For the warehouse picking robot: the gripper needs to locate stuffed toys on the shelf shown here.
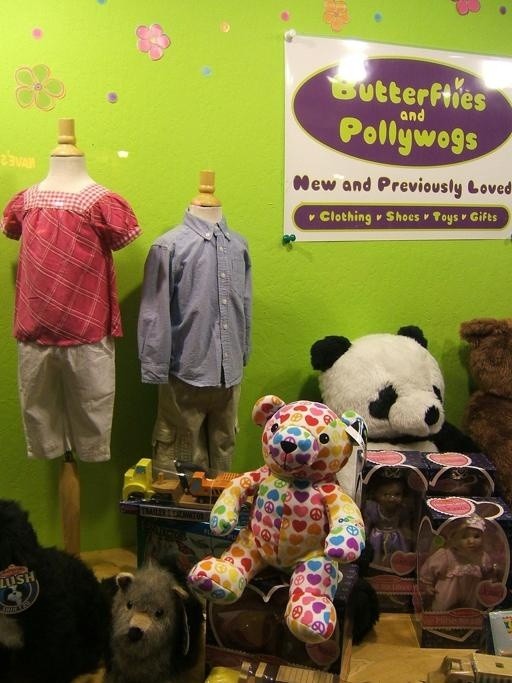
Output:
[97,560,206,682]
[308,324,479,458]
[458,319,510,522]
[0,500,110,683]
[186,395,367,646]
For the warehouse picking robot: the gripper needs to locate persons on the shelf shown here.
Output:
[356,448,512,643]
[2,120,141,557]
[139,170,253,483]
